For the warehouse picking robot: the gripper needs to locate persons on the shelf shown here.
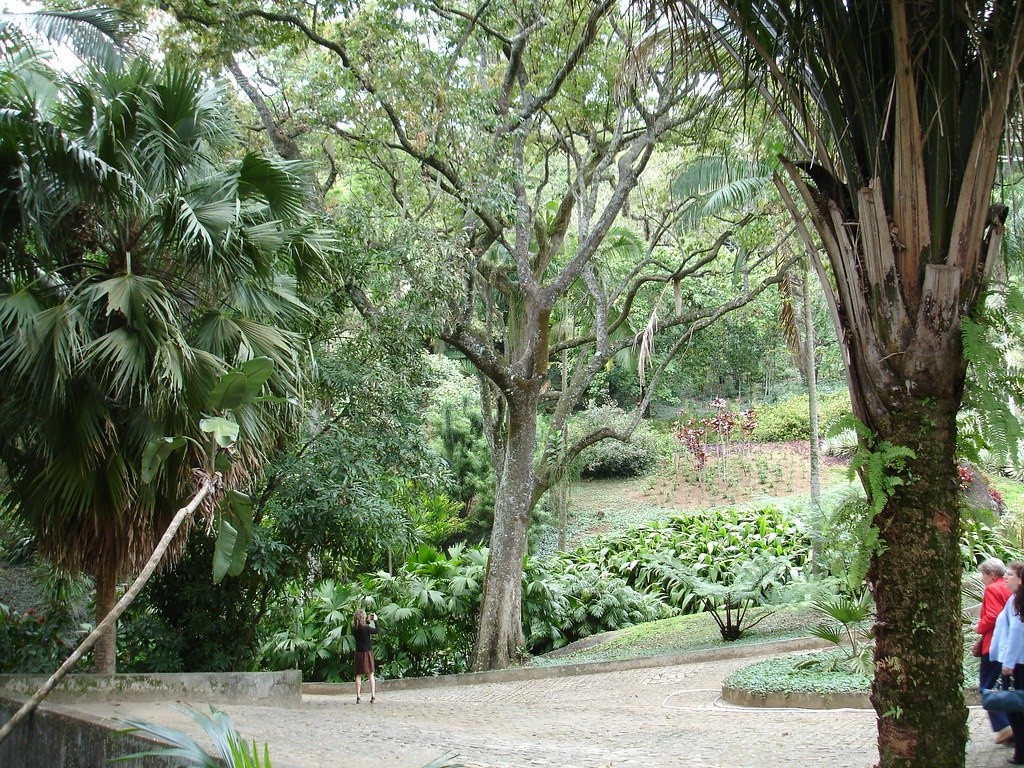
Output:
[351,609,380,705]
[982,690,1024,764]
[988,561,1024,747]
[972,558,1014,712]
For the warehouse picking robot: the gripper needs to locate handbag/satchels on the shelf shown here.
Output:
[973,636,982,657]
[979,674,1024,712]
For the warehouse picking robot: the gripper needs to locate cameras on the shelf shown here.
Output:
[371,615,374,620]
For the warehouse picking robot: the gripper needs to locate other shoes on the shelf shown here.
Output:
[357,697,362,704]
[1007,756,1024,764]
[370,697,375,703]
[995,726,1013,744]
